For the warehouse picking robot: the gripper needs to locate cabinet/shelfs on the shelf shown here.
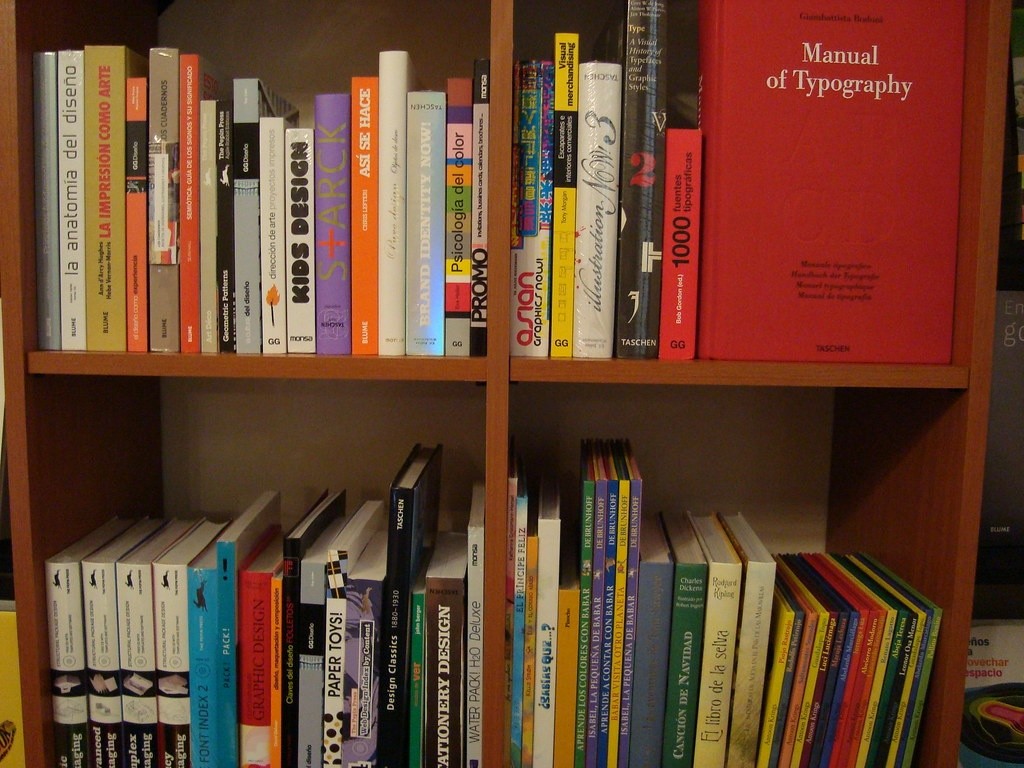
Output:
[0,0,1016,768]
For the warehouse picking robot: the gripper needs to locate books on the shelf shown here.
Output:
[628,509,778,768]
[44,487,388,768]
[576,440,642,768]
[697,1,967,363]
[31,45,314,354]
[507,434,580,768]
[514,33,621,360]
[956,620,1024,768]
[381,442,484,768]
[615,0,702,359]
[313,50,491,356]
[756,549,943,768]
[0,600,27,767]
